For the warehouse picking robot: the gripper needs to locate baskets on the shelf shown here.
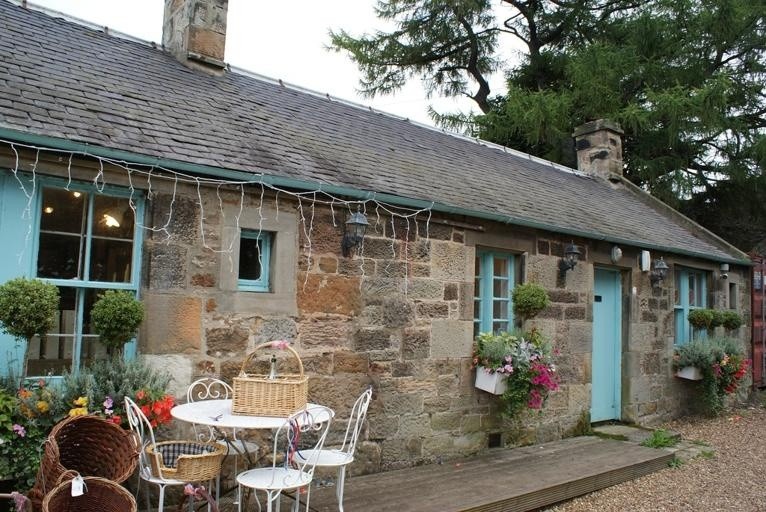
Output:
[232,342,308,417]
[26,414,141,512]
[146,440,226,481]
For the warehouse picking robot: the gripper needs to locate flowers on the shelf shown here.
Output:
[471,333,562,419]
[674,337,752,415]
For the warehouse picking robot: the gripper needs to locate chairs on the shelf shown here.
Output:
[282,388,374,512]
[125,395,221,511]
[235,405,333,512]
[187,378,259,504]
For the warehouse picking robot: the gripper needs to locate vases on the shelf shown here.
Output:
[475,364,509,395]
[675,366,705,381]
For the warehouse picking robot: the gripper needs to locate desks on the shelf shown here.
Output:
[170,398,335,512]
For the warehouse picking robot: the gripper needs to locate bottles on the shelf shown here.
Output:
[267,354,279,379]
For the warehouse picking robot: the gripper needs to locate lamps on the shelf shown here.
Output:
[640,249,650,273]
[103,200,132,229]
[612,245,623,264]
[651,257,669,286]
[341,211,369,258]
[719,264,730,280]
[561,240,581,276]
